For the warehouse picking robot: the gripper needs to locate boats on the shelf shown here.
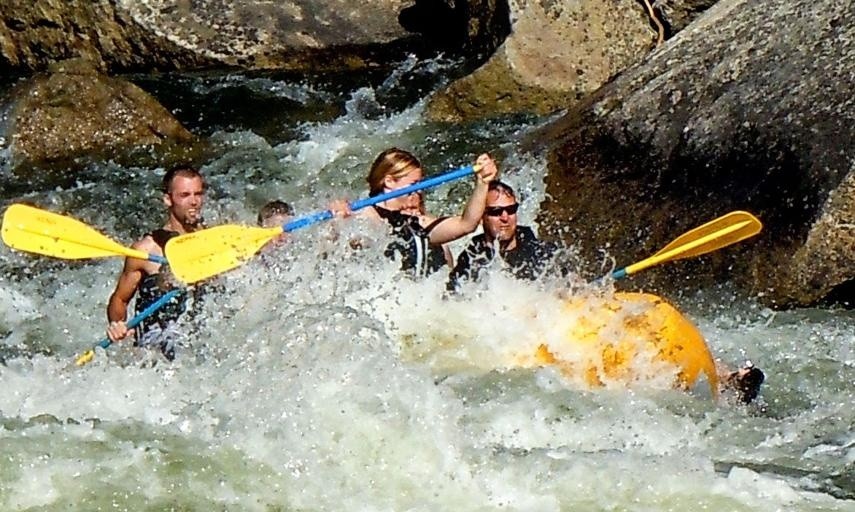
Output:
[417,292,717,405]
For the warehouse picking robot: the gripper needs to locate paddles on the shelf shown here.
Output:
[1,204,168,265]
[587,212,762,289]
[165,162,485,282]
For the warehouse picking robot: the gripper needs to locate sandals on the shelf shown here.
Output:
[724,364,764,403]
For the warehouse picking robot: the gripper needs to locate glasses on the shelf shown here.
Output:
[485,201,519,216]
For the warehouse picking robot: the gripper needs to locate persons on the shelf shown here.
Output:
[325,148,492,283]
[106,167,208,345]
[719,364,764,410]
[256,199,294,255]
[447,179,572,298]
